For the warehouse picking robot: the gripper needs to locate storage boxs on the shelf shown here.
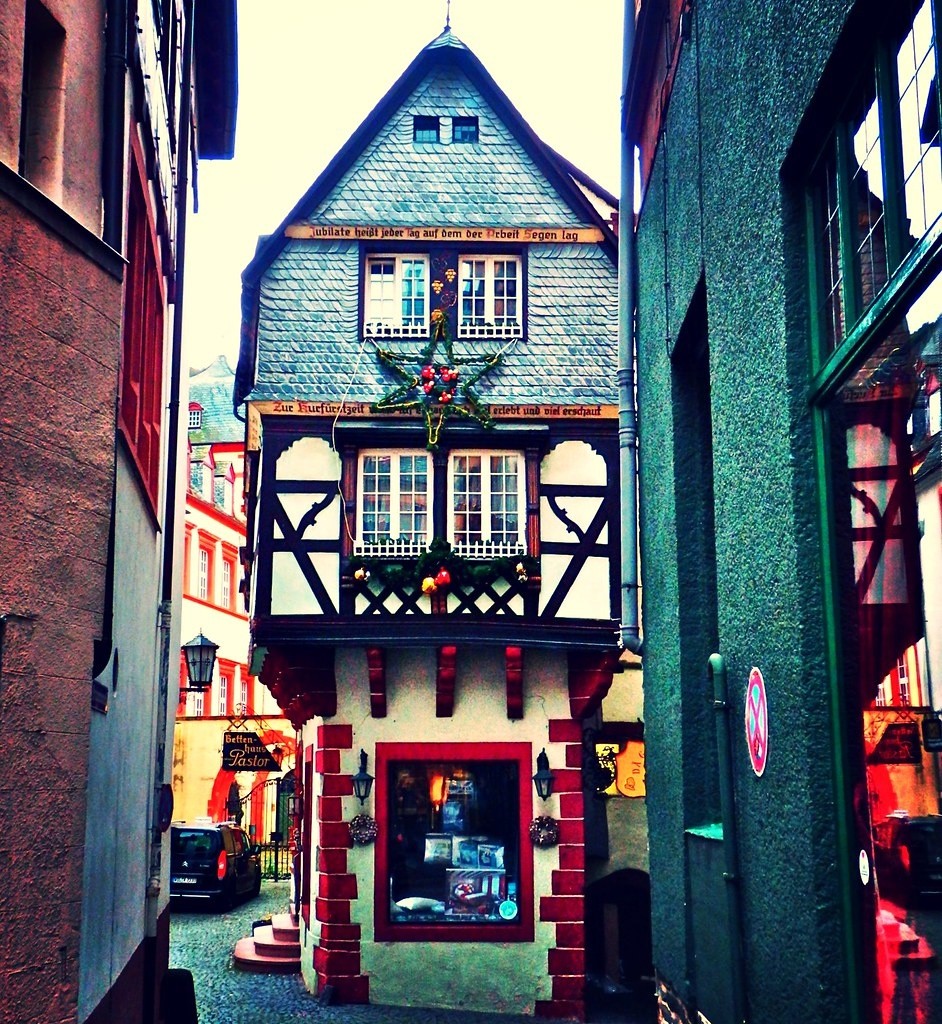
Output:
[424,833,509,920]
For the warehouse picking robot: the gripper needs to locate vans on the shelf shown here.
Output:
[171,822,261,913]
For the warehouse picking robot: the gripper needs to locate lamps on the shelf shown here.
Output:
[350,750,376,806]
[180,628,221,694]
[287,792,304,816]
[533,747,556,800]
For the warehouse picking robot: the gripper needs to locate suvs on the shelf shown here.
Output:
[872,812,942,909]
